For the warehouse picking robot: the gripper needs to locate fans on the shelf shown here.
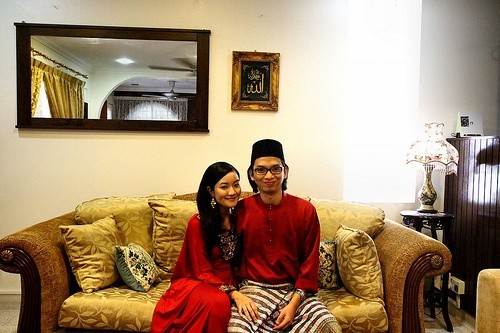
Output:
[149,66,197,76]
[142,81,178,100]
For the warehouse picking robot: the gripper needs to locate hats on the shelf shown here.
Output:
[251,139,284,162]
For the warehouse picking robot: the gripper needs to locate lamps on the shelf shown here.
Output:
[406,122,459,213]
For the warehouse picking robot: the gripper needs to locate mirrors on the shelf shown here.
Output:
[14,23,210,133]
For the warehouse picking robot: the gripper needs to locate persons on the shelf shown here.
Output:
[228,139,342,333]
[151,162,260,333]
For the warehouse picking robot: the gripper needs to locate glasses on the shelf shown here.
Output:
[252,166,285,175]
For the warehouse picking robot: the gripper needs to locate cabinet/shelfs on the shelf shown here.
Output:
[442,136,500,328]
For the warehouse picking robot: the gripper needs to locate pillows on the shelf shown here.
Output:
[148,199,199,278]
[114,242,161,293]
[318,242,339,290]
[75,192,176,256]
[334,224,385,303]
[59,214,123,293]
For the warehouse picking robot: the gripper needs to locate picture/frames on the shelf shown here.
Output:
[231,50,280,111]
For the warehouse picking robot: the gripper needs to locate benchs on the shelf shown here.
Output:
[0,191,452,333]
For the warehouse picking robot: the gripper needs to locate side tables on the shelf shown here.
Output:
[401,210,455,333]
